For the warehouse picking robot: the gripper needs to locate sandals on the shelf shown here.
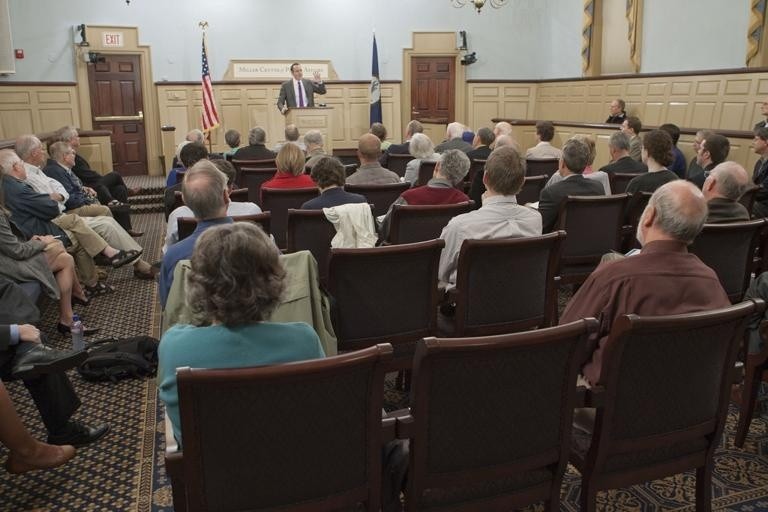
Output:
[107,201,130,213]
[108,249,143,268]
[85,281,116,297]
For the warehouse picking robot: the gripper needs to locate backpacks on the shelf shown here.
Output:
[77,337,160,381]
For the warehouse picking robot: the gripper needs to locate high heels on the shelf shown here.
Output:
[58,320,101,337]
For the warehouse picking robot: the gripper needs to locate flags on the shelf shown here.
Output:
[370,35,382,125]
[200,34,221,141]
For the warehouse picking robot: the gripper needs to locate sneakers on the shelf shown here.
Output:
[134,264,160,280]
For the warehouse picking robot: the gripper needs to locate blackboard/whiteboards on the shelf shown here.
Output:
[0,0,16,74]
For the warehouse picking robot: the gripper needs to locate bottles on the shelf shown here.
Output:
[70,315,83,354]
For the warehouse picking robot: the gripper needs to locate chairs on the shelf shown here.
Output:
[18,282,42,303]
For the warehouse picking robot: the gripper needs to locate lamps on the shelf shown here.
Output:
[451,0,509,13]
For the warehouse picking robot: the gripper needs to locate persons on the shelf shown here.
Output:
[157,123,324,447]
[540,116,767,434]
[0,126,160,475]
[605,99,626,124]
[277,62,327,115]
[301,134,399,211]
[373,120,563,315]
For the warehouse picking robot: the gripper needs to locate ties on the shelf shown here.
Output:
[298,82,306,108]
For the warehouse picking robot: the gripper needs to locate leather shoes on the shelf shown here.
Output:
[12,343,88,378]
[72,295,91,307]
[6,443,75,474]
[128,229,143,237]
[48,421,110,447]
